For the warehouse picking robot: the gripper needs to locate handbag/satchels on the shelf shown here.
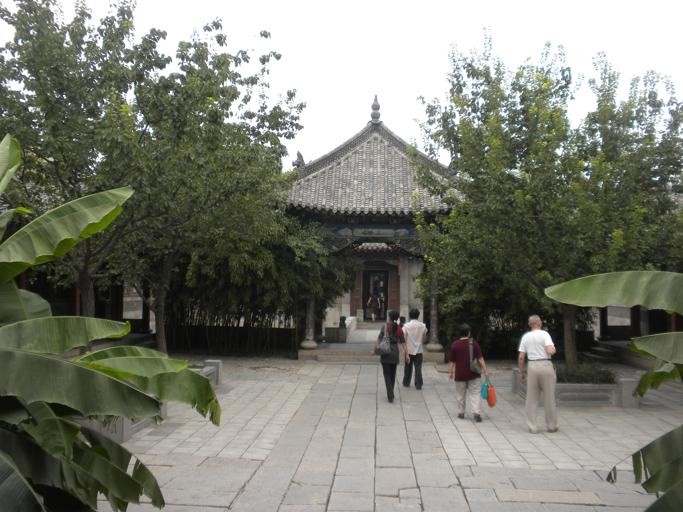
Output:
[374,323,390,355]
[470,361,496,406]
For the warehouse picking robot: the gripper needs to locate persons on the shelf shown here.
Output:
[367,290,381,322]
[446,324,489,422]
[517,314,559,434]
[376,308,428,404]
